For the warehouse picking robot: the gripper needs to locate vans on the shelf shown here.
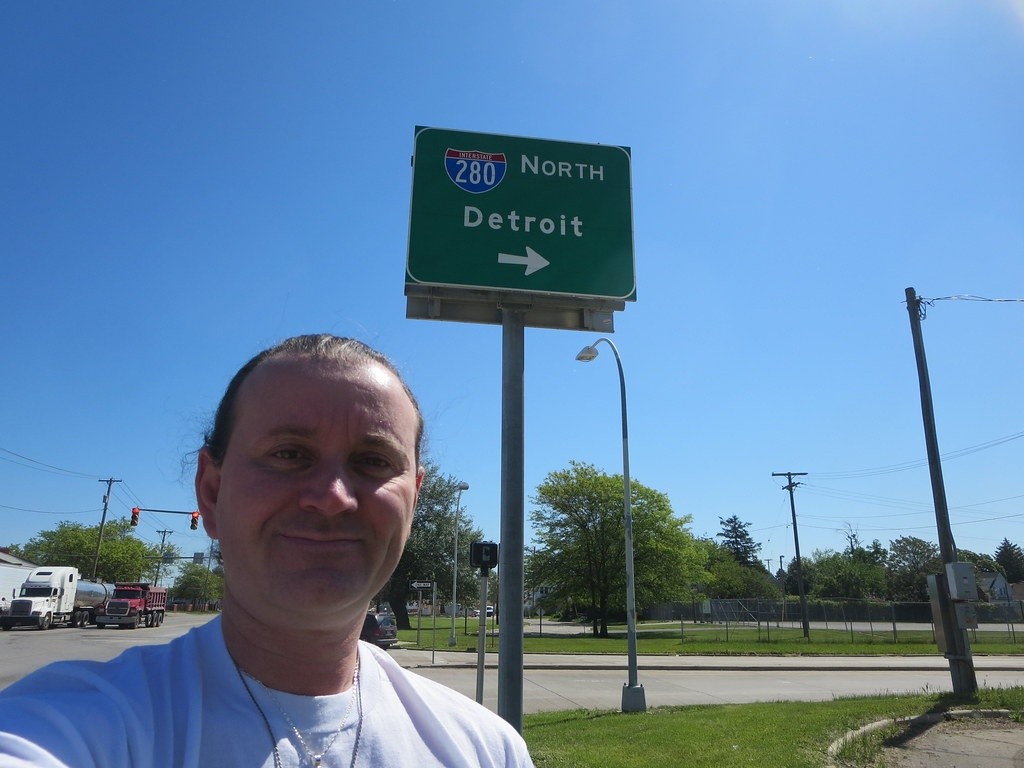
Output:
[487,606,494,616]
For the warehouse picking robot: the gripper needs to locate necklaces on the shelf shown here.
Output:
[227,646,364,768]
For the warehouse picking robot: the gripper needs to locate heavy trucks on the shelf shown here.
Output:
[95,582,166,629]
[0,566,117,631]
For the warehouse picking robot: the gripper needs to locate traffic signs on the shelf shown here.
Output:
[409,579,435,591]
[405,123,637,303]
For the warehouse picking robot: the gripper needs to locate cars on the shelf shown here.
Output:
[471,610,480,617]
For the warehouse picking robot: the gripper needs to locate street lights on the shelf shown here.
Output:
[574,337,649,715]
[449,481,470,646]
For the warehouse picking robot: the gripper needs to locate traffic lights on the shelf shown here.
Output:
[190,511,200,530]
[130,507,140,526]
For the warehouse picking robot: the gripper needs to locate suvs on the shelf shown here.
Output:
[360,612,399,650]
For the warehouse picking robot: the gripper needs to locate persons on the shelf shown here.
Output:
[0,333,535,768]
